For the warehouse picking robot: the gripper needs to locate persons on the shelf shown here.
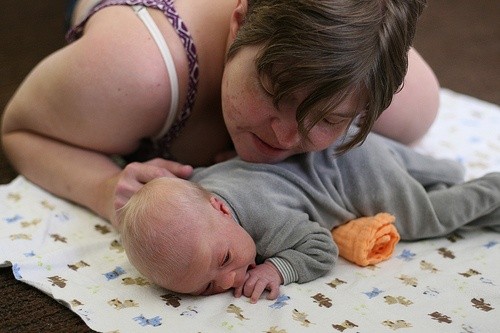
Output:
[0,0,440,227]
[115,126,500,304]
